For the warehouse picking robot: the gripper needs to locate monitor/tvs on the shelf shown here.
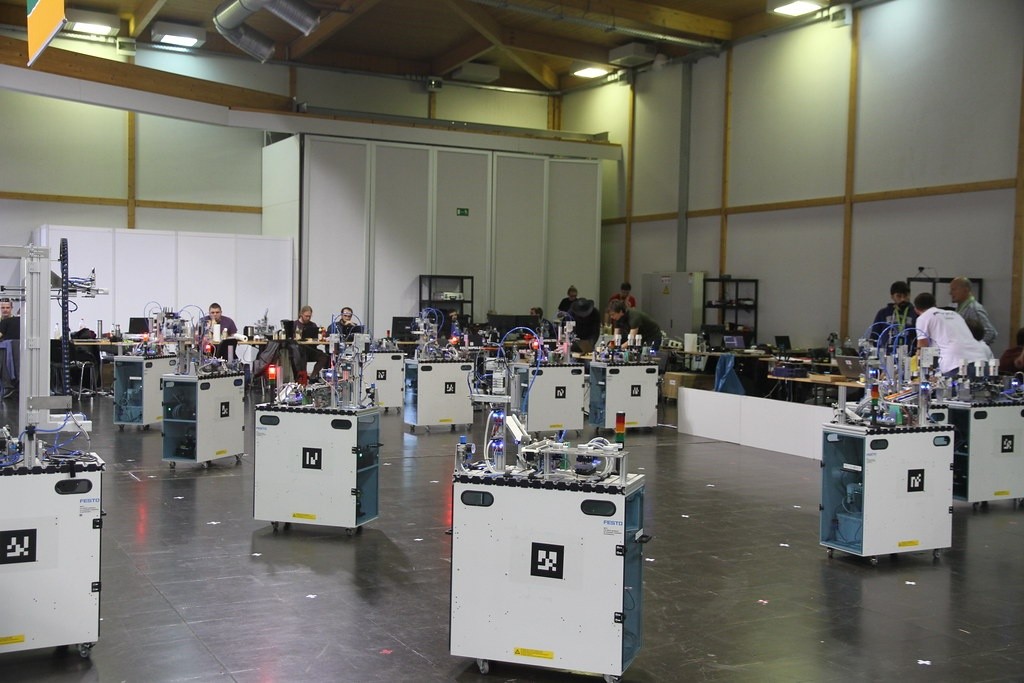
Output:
[342,326,364,341]
[515,315,540,336]
[392,317,415,341]
[775,336,791,350]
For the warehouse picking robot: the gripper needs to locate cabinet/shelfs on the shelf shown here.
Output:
[932,398,1024,509]
[0,348,663,683]
[819,420,955,569]
[418,275,474,333]
[701,279,760,350]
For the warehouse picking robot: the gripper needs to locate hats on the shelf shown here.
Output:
[570,297,594,317]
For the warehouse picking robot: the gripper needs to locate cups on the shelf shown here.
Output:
[244,325,254,340]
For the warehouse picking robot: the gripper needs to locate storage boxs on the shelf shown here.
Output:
[664,371,714,399]
[836,513,862,545]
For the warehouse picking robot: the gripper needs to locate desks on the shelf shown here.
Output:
[75,342,137,356]
[299,341,352,345]
[574,353,594,360]
[237,341,267,347]
[394,340,418,345]
[676,351,771,372]
[505,339,528,347]
[767,375,866,405]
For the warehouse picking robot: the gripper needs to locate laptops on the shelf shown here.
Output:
[298,327,319,341]
[835,355,865,380]
[125,318,152,334]
[724,336,746,350]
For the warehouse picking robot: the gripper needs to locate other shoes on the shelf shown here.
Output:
[310,373,319,379]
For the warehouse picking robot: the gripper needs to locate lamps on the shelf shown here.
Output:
[63,8,120,37]
[150,21,208,49]
[764,0,830,19]
[557,61,613,81]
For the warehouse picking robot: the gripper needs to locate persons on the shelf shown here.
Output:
[0,297,20,399]
[326,307,357,367]
[559,285,600,338]
[196,303,237,357]
[870,276,1024,387]
[604,282,663,351]
[294,306,329,384]
[530,307,557,351]
[449,310,468,337]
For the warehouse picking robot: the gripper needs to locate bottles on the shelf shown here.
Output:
[295,325,301,339]
[96,319,102,338]
[386,330,391,341]
[53,323,60,339]
[110,324,121,339]
[273,328,286,340]
[79,318,85,330]
[318,325,326,341]
[221,327,228,339]
